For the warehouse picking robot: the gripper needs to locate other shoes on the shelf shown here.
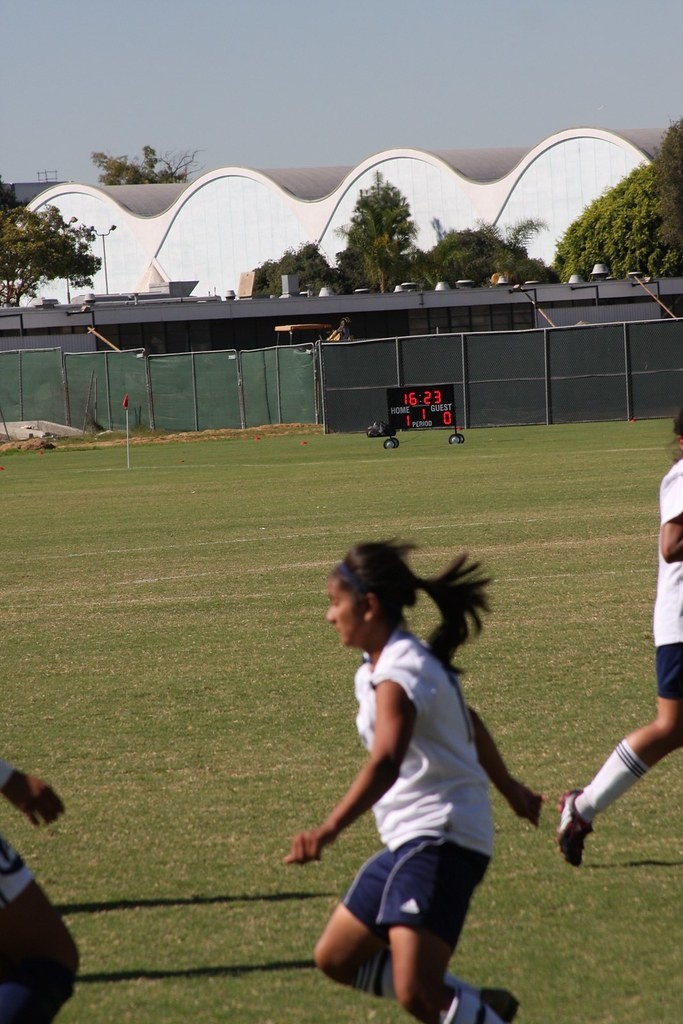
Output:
[480,984,519,1024]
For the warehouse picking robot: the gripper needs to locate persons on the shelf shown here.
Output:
[555,406,683,866]
[283,535,542,1024]
[0,751,81,1024]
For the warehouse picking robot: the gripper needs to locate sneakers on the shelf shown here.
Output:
[556,789,594,867]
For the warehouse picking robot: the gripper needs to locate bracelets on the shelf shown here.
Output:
[492,761,512,792]
